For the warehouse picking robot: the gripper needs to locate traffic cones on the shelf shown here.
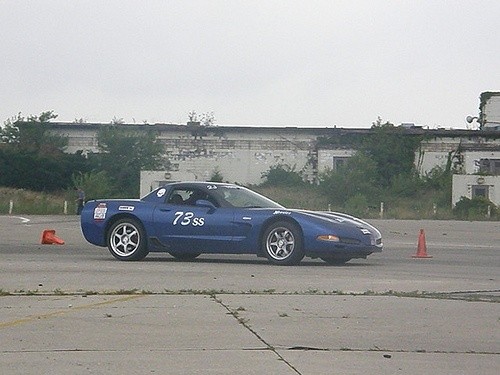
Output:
[40,229,65,246]
[410,228,433,259]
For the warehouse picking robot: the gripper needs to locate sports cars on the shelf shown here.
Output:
[81,181,385,266]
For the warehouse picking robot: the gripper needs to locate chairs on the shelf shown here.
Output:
[169,189,226,207]
